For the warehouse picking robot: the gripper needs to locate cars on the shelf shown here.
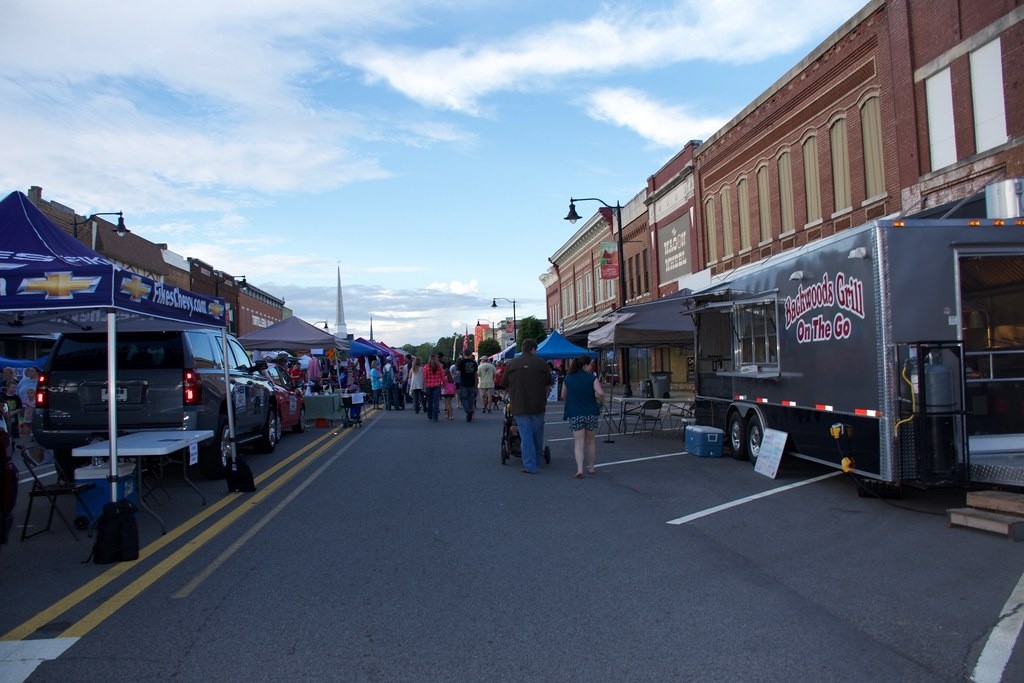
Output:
[261,363,306,443]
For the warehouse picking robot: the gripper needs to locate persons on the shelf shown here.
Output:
[547,362,565,400]
[498,338,554,474]
[319,348,509,423]
[560,355,605,478]
[0,367,39,457]
[286,362,305,389]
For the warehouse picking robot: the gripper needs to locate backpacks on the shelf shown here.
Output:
[382,365,395,388]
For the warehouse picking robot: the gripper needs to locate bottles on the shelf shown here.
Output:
[91,436,101,466]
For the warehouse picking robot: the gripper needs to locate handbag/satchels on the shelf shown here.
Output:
[409,387,415,400]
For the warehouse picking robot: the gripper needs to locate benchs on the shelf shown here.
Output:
[66,347,129,369]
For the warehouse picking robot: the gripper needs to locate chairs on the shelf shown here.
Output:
[16,444,101,542]
[599,400,695,438]
[163,346,184,366]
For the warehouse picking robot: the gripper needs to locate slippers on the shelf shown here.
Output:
[575,473,584,478]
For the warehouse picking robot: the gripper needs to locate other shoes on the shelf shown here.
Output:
[372,405,383,410]
[427,414,439,421]
[482,408,492,414]
[415,407,428,414]
[467,411,474,421]
[388,405,402,412]
[447,415,454,419]
[521,467,526,472]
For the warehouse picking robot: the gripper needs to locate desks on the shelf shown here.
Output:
[72,430,215,536]
[605,397,695,434]
[339,404,364,429]
[302,392,367,426]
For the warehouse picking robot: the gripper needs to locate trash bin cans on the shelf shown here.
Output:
[651,371,672,398]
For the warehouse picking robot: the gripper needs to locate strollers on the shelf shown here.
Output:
[501,402,551,465]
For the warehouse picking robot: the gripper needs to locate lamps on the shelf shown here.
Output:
[969,308,985,329]
[847,246,866,259]
[789,271,804,281]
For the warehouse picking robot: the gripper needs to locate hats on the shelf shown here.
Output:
[437,352,446,357]
[480,355,489,360]
[387,356,393,360]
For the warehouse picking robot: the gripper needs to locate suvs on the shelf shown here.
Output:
[31,323,280,480]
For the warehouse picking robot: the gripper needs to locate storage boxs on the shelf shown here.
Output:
[74,462,141,529]
[684,425,724,458]
[682,421,695,443]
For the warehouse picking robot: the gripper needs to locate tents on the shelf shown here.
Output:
[514,330,599,379]
[311,337,405,404]
[1,189,238,502]
[587,305,695,443]
[235,316,366,421]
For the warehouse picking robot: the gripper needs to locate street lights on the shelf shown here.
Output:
[72,211,132,242]
[564,198,633,399]
[476,319,494,339]
[490,297,516,342]
[215,275,249,298]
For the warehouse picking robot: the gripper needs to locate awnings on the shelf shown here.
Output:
[547,307,613,338]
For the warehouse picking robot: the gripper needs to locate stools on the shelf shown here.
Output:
[365,393,374,405]
